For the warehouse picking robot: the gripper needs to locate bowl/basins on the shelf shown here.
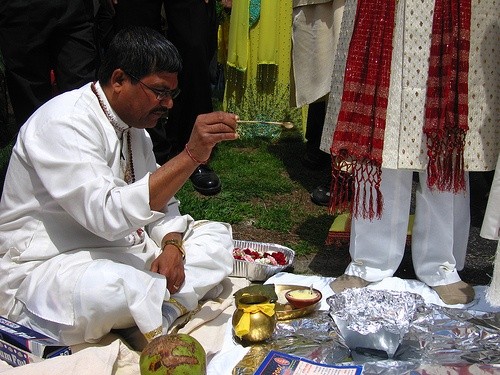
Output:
[285,288,321,308]
[233,284,322,320]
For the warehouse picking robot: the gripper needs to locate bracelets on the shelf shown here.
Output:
[162,240,186,261]
[185,143,208,166]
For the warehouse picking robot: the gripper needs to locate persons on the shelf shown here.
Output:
[1,25,239,351]
[319,1,500,305]
[0,1,500,215]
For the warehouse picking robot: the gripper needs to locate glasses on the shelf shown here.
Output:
[124,70,181,101]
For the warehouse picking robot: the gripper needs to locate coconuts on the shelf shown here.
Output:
[139,309,208,375]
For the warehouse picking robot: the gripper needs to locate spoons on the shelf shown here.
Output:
[236,121,293,128]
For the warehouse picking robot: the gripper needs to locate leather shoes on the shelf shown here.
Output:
[189,162,222,194]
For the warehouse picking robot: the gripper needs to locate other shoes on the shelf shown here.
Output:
[313,176,350,206]
